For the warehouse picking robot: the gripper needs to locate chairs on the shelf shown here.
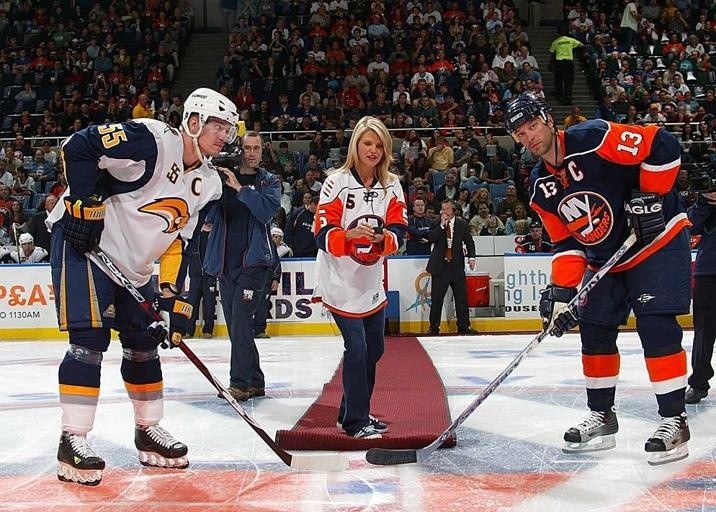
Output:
[1,38,58,232]
[291,140,515,201]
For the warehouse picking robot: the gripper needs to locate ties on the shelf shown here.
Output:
[446,221,452,260]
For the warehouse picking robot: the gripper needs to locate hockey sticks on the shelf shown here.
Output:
[366,232,637,466]
[92,243,350,471]
[13,223,21,264]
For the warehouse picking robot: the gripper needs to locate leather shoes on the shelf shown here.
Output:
[458,328,476,333]
[428,329,438,334]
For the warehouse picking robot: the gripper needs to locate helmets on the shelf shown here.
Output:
[182,87,239,127]
[19,233,33,247]
[504,94,551,135]
[270,227,284,240]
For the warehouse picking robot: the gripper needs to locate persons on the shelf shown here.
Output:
[504,94,692,452]
[201,130,283,402]
[312,115,409,440]
[252,242,281,339]
[44,88,239,470]
[183,210,215,339]
[685,192,716,404]
[425,198,479,336]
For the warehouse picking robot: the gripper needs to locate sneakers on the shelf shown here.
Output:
[337,414,388,439]
[564,407,618,442]
[57,430,105,469]
[135,424,188,458]
[685,387,708,403]
[645,412,690,451]
[218,387,265,400]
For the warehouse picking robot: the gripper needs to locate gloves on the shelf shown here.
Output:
[631,187,665,242]
[63,196,105,254]
[144,292,194,347]
[540,283,578,338]
